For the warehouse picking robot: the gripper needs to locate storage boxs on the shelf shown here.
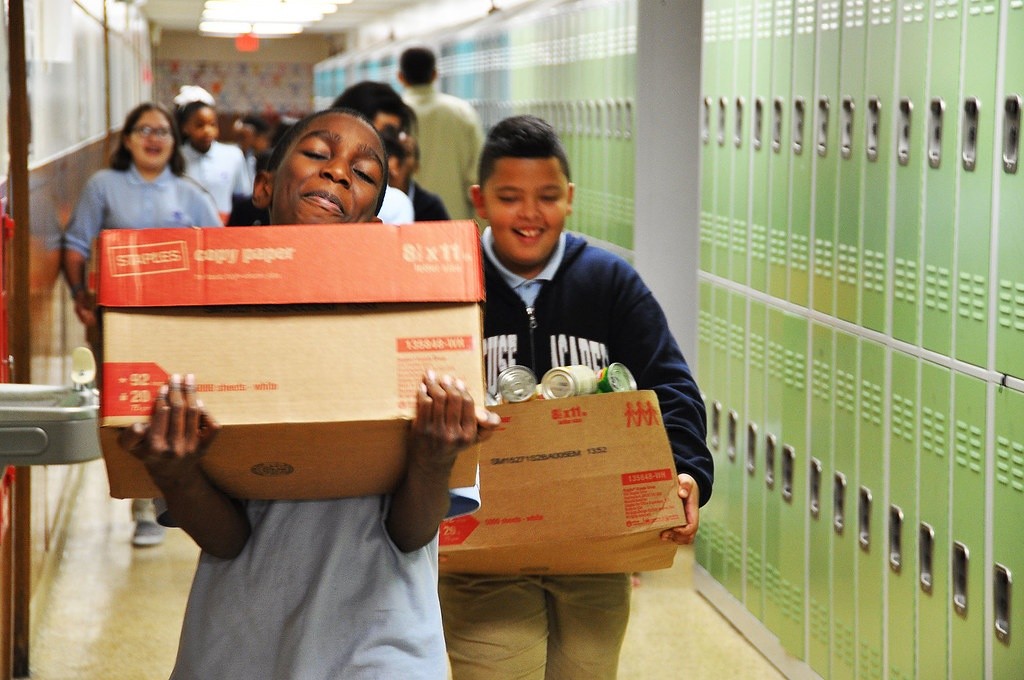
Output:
[85,219,483,500]
[437,389,686,577]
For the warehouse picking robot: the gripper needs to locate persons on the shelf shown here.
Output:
[61,102,228,546]
[230,81,453,225]
[116,106,502,680]
[392,47,485,224]
[170,80,251,227]
[437,115,717,680]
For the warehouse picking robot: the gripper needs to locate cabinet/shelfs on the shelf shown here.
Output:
[690,0,1024,680]
[502,1,637,267]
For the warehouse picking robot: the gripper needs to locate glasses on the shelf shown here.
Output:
[132,125,171,140]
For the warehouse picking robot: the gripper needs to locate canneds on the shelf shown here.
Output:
[496,361,637,404]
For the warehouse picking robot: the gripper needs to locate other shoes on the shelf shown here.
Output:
[132,521,165,546]
